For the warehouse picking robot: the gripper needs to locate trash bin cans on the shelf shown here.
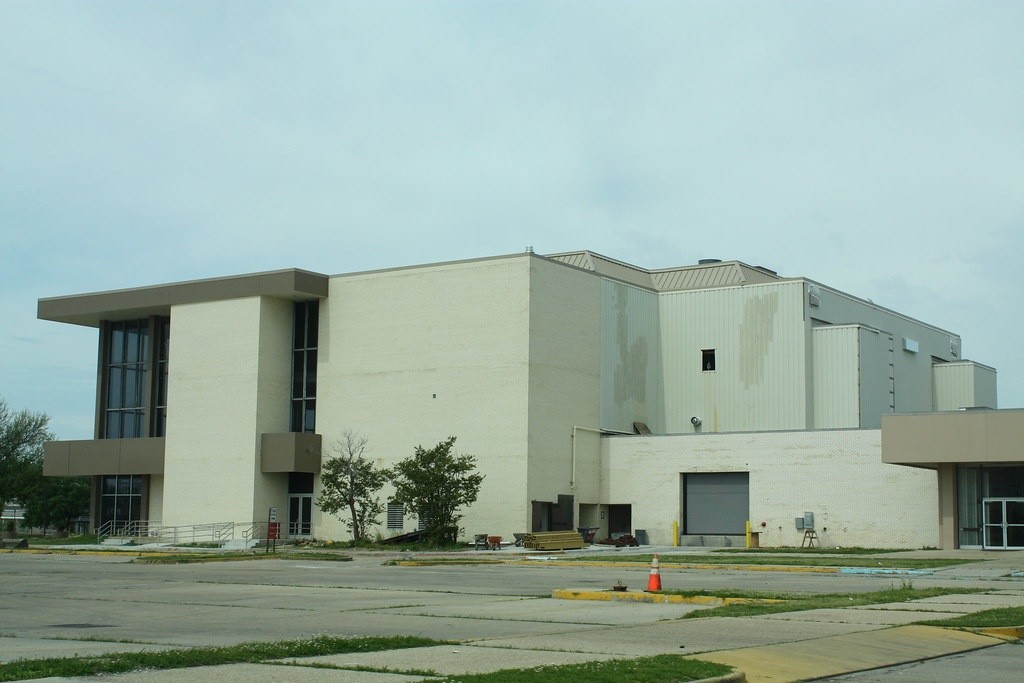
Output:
[269,522,281,539]
[635,529,645,544]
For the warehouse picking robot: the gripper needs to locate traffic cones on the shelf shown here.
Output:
[644,554,662,592]
